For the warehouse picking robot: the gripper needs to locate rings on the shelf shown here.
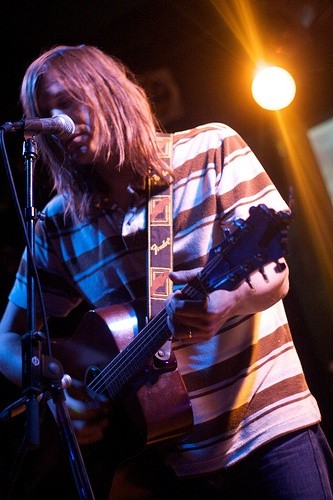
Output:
[189,329,192,339]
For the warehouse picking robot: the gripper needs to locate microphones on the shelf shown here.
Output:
[1,114,75,140]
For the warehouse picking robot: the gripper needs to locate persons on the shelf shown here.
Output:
[0,45,333,500]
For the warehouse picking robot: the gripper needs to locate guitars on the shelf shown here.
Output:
[0,204,294,500]
